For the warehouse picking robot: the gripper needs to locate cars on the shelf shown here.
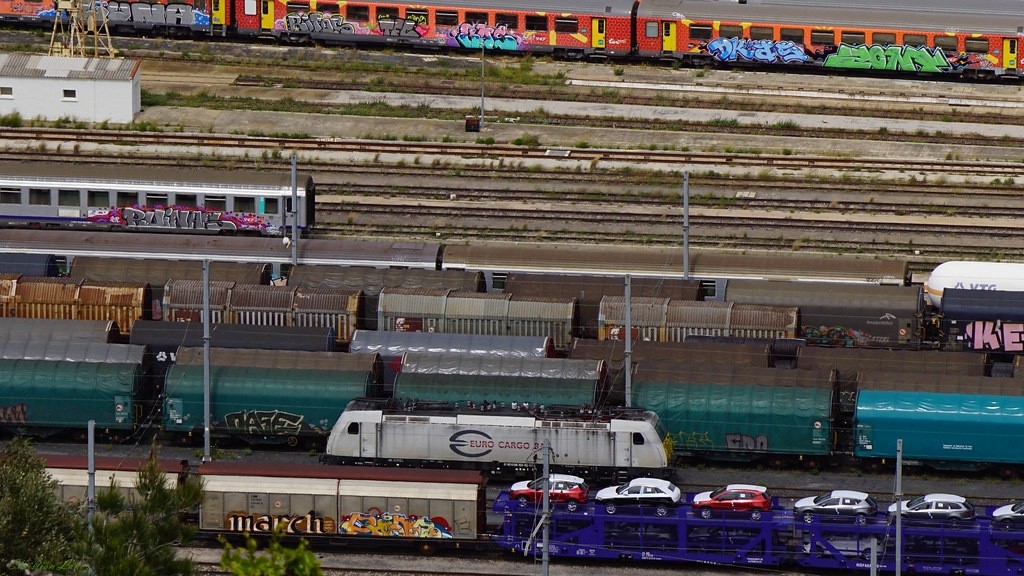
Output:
[803,533,885,562]
[605,522,673,547]
[594,477,681,517]
[992,500,1024,533]
[793,490,878,525]
[688,526,762,555]
[692,484,773,521]
[518,516,579,543]
[905,535,978,566]
[510,474,589,512]
[886,494,976,530]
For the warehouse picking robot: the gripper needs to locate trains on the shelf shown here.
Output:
[0,159,1024,576]
[0,0,1024,85]
